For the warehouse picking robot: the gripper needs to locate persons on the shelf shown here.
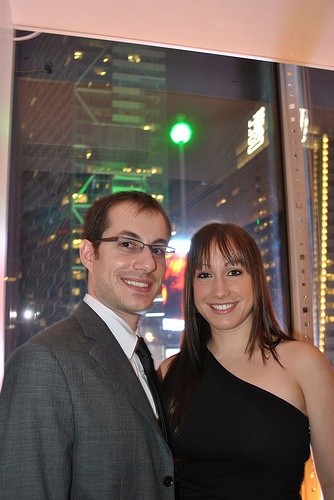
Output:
[0,190,173,499]
[157,223,334,500]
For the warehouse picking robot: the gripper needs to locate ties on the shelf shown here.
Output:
[135,337,168,444]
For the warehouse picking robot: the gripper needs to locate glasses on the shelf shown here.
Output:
[90,236,176,260]
